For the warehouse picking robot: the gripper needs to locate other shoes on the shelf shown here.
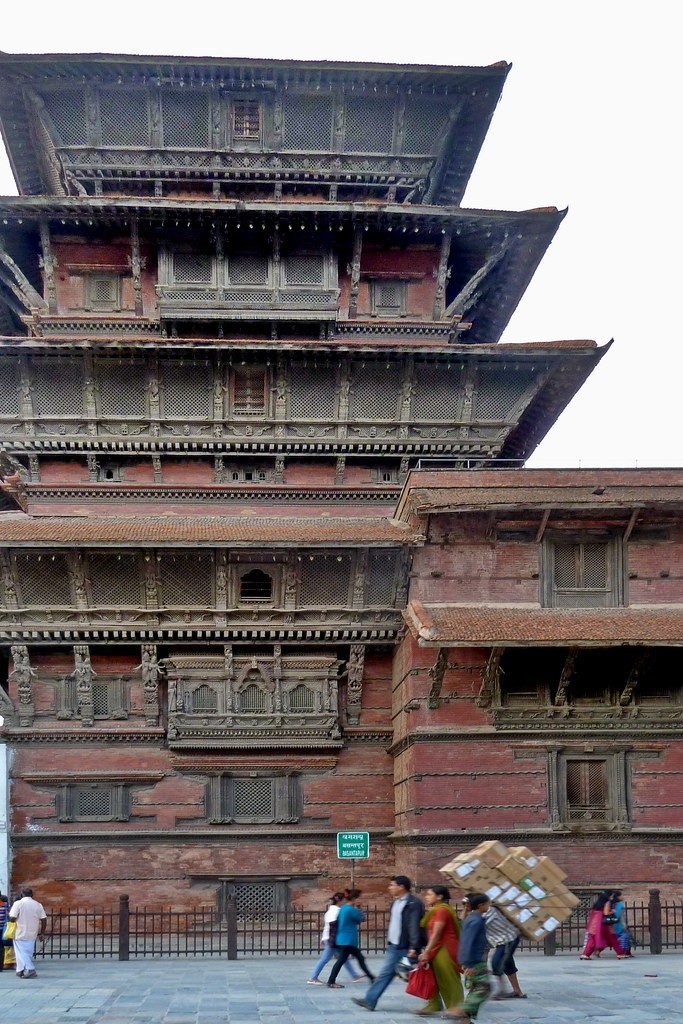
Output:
[499,993,528,1000]
[306,979,326,986]
[442,1008,475,1024]
[15,971,23,977]
[353,975,367,982]
[327,983,345,988]
[580,955,590,960]
[617,954,631,959]
[413,1006,439,1015]
[349,998,375,1012]
[21,969,36,978]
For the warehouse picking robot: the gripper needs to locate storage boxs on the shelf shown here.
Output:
[439,840,580,943]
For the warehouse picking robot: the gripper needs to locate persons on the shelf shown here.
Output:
[135,653,165,686]
[459,895,527,1000]
[446,893,492,1024]
[397,568,408,595]
[426,651,448,686]
[356,562,366,591]
[66,556,91,590]
[560,655,580,681]
[580,890,632,960]
[17,373,478,401]
[348,655,364,688]
[216,569,228,590]
[307,888,378,988]
[9,653,39,686]
[34,228,452,285]
[0,558,15,593]
[9,888,49,979]
[480,656,501,695]
[139,564,162,588]
[417,885,465,1019]
[73,655,97,686]
[0,891,15,972]
[287,565,298,589]
[351,876,425,1011]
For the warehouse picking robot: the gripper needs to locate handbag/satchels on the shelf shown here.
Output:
[3,921,16,938]
[406,961,437,1000]
[328,921,339,949]
[602,913,619,924]
